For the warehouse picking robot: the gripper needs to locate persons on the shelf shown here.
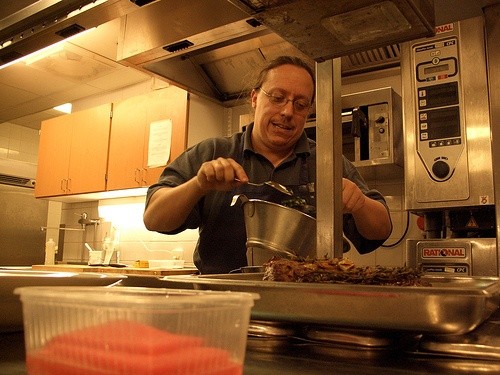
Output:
[143,55,393,275]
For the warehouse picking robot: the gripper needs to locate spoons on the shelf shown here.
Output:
[233,177,295,196]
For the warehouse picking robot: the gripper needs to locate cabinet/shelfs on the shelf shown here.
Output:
[36,85,231,204]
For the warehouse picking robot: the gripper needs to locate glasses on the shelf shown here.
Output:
[259,86,311,111]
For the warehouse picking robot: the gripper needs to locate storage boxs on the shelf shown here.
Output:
[15,285,260,375]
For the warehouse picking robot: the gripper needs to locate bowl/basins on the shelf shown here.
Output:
[230,192,351,266]
